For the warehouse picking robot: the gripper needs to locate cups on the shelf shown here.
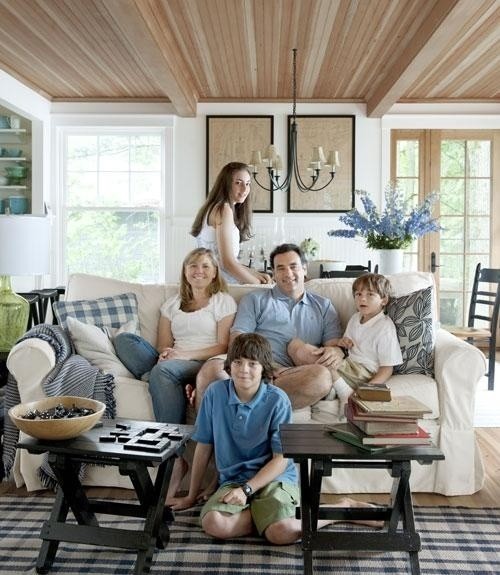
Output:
[7,114,21,130]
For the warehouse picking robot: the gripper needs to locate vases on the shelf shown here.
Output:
[0,274,30,353]
[377,249,404,273]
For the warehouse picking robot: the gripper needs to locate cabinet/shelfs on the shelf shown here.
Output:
[1,106,45,219]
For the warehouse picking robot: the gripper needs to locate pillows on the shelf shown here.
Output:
[382,286,438,375]
[48,293,140,346]
[66,316,135,382]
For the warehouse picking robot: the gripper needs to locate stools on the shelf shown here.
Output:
[33,289,58,326]
[16,293,40,331]
[57,286,65,294]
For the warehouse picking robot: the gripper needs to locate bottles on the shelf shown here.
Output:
[274,218,286,245]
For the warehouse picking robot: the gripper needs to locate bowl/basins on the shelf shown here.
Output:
[6,393,108,441]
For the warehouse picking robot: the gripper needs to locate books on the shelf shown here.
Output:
[325,381,433,455]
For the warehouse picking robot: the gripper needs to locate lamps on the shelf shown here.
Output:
[250,49,339,193]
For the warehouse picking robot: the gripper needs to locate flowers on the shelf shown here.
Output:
[327,180,445,249]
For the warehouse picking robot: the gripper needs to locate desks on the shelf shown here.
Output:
[278,423,445,575]
[14,416,196,575]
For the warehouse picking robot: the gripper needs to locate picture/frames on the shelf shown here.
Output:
[207,114,274,212]
[288,115,355,213]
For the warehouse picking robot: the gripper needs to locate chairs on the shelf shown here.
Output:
[318,264,378,278]
[440,263,500,392]
[346,260,372,271]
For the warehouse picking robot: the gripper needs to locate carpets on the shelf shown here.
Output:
[0,492,500,575]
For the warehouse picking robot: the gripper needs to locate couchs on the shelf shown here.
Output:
[7,271,487,496]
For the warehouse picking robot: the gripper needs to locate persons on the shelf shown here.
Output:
[286,272,403,423]
[191,162,272,285]
[165,333,385,545]
[195,244,342,506]
[113,247,237,502]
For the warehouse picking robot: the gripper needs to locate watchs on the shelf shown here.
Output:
[243,483,253,496]
[340,346,349,360]
